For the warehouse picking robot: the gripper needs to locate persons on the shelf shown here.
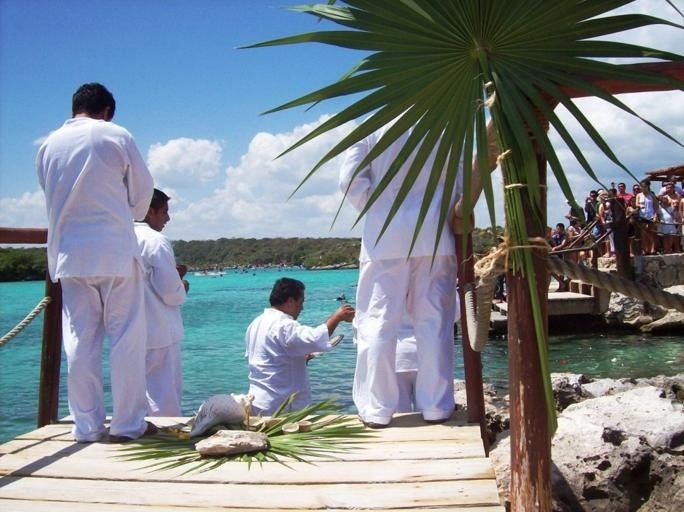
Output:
[129,186,190,417]
[32,81,157,444]
[338,105,465,430]
[494,180,684,302]
[244,275,356,418]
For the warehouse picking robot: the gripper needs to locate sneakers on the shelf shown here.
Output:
[107,421,157,442]
